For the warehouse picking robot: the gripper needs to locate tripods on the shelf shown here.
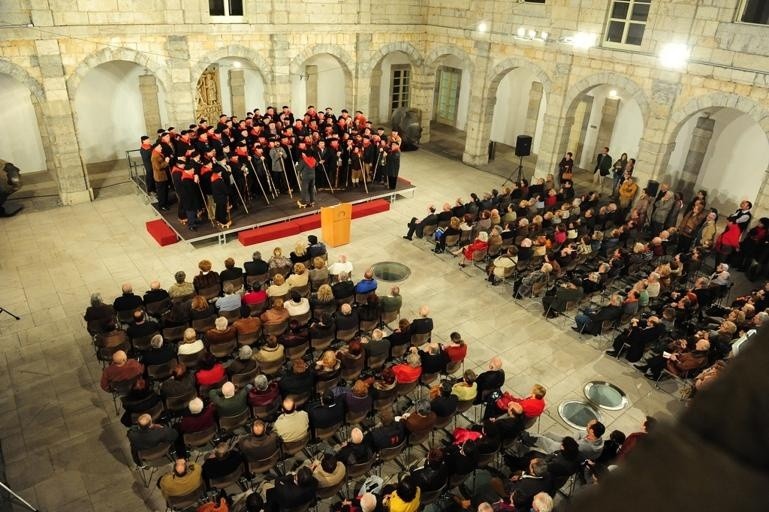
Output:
[502,157,525,187]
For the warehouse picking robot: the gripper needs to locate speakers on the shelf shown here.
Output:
[515,135,532,156]
[647,180,659,197]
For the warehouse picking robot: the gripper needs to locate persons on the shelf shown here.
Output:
[609,153,627,197]
[589,147,612,198]
[570,245,769,405]
[85,234,433,457]
[619,159,635,189]
[406,174,768,319]
[558,152,573,189]
[157,330,656,511]
[141,106,402,230]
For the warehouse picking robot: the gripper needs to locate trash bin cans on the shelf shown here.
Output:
[488,141,497,161]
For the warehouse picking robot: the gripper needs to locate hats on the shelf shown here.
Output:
[140,106,399,173]
